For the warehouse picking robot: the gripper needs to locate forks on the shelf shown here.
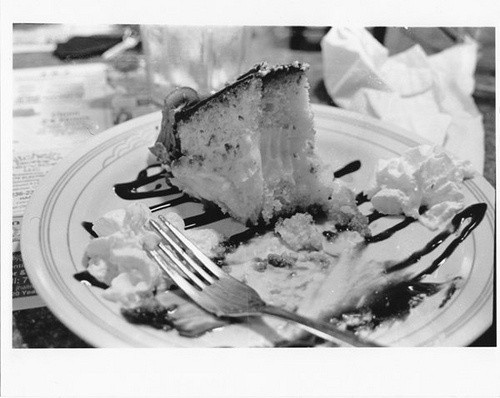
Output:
[142,217,389,349]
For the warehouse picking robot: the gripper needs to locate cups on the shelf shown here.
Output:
[140,26,292,101]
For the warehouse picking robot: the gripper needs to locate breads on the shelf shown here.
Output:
[168,60,328,227]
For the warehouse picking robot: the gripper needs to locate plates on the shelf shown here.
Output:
[19,103,497,347]
